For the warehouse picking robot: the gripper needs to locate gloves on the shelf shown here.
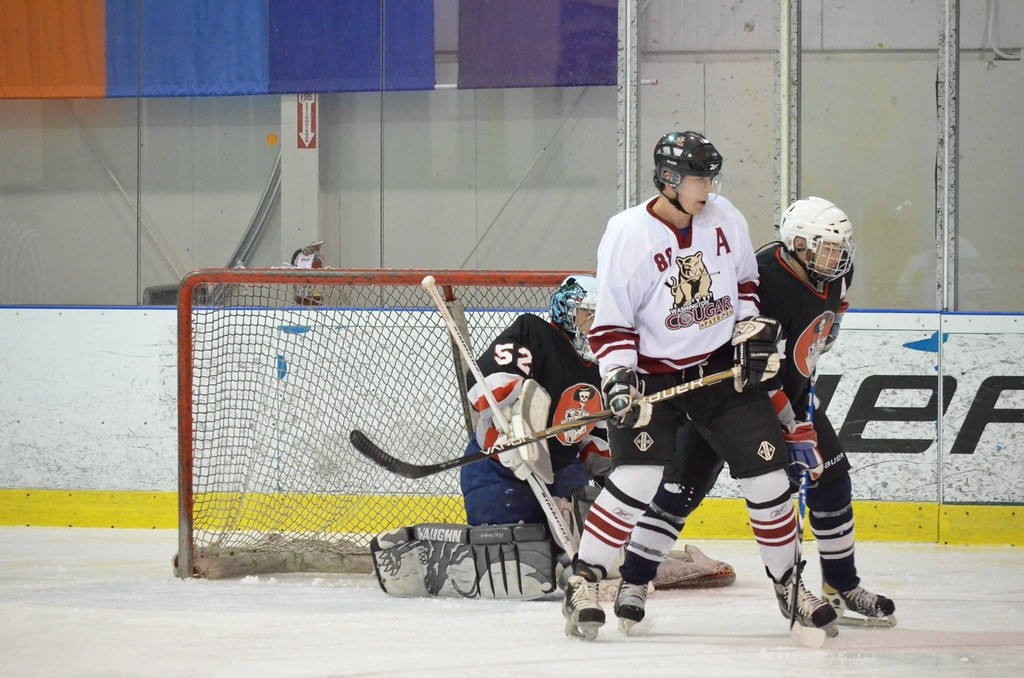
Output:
[732,315,787,393]
[781,420,824,489]
[603,369,654,430]
[820,301,848,354]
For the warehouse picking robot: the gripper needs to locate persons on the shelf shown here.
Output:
[556,128,839,630]
[613,192,896,622]
[369,273,736,602]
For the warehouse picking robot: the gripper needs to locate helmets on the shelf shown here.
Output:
[654,130,723,186]
[780,196,856,283]
[548,274,598,342]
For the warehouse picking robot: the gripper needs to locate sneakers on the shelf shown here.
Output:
[821,586,896,628]
[563,575,605,641]
[765,560,839,638]
[614,578,648,634]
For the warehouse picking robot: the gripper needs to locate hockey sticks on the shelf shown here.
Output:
[350,365,743,480]
[790,378,826,649]
[422,275,656,602]
